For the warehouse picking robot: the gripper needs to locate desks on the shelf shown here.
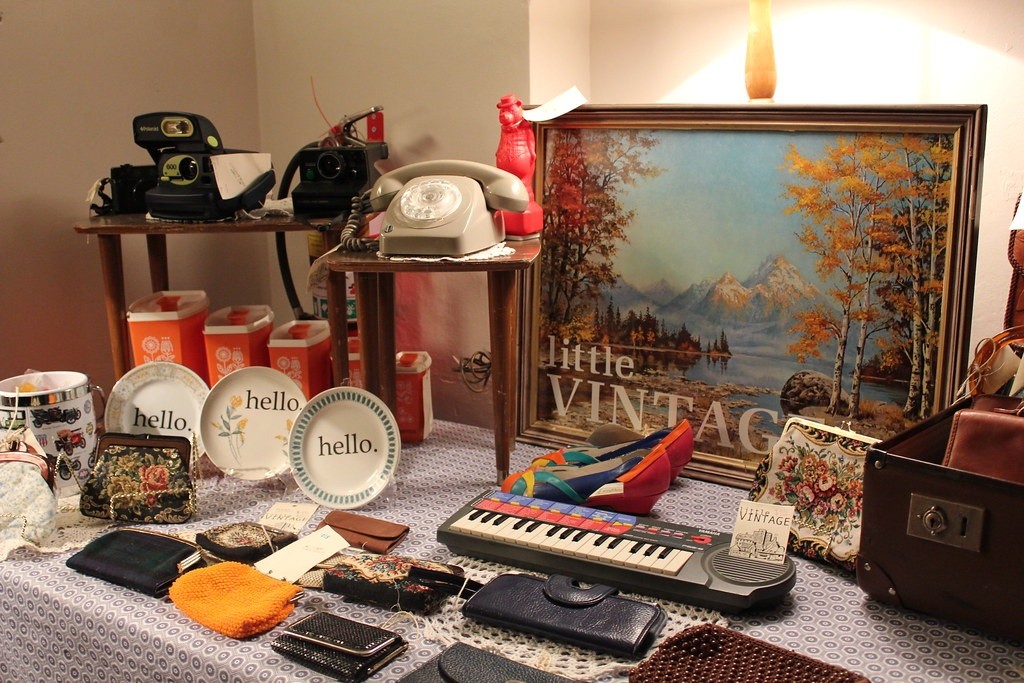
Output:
[76,214,397,413]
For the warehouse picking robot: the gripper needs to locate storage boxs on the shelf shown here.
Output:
[396,350,437,441]
[855,394,1024,642]
[267,319,332,400]
[126,289,211,389]
[347,337,362,388]
[204,305,276,388]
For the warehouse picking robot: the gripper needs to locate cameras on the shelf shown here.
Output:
[291,141,389,218]
[111,163,160,214]
[133,112,276,222]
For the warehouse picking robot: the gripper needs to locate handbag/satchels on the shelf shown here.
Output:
[943,401,1024,485]
[80,433,192,524]
[748,418,883,573]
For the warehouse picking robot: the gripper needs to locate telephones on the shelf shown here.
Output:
[372,157,530,258]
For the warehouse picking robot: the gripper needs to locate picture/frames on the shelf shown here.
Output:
[515,105,990,493]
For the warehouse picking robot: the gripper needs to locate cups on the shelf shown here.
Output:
[0,372,105,498]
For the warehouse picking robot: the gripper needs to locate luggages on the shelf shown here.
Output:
[858,392,1024,646]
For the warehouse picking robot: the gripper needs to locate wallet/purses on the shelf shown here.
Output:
[67,510,870,683]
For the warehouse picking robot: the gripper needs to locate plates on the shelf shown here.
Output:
[104,362,209,465]
[289,387,401,510]
[201,367,308,480]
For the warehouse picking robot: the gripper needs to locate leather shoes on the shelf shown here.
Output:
[501,419,693,516]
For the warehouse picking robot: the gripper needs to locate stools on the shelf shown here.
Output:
[326,236,544,488]
